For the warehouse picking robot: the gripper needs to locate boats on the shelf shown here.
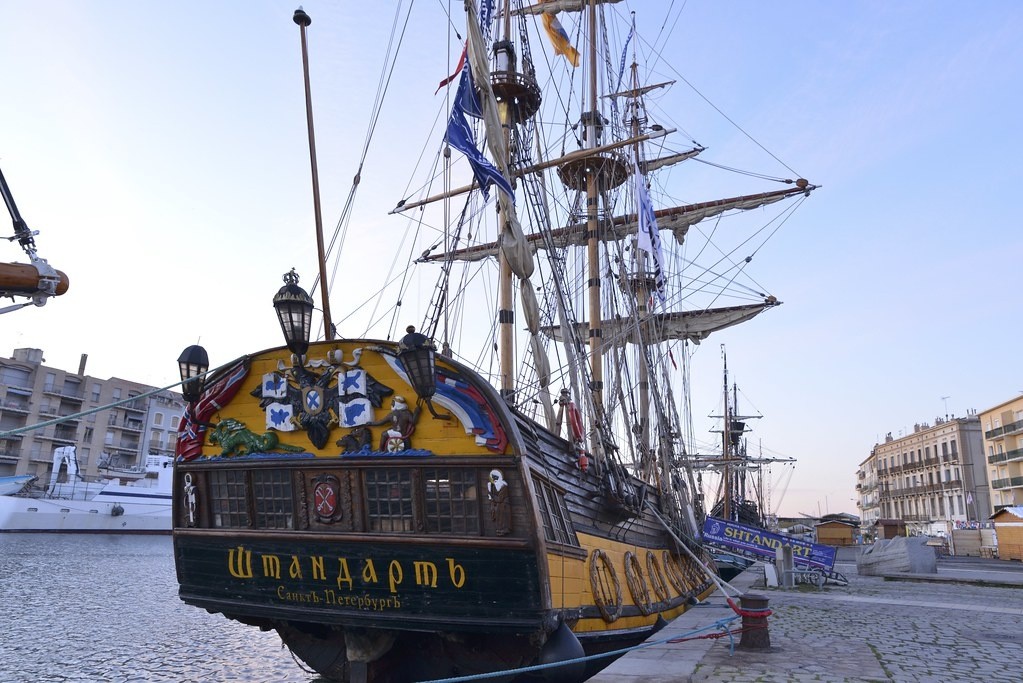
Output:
[0,445,175,533]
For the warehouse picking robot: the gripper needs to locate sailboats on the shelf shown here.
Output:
[171,0,824,683]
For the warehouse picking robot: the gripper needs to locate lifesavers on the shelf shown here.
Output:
[589,550,624,622]
[644,549,708,606]
[570,401,587,441]
[624,550,652,615]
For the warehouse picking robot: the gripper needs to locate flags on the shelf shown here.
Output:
[635,166,667,300]
[435,0,516,205]
[538,0,580,67]
